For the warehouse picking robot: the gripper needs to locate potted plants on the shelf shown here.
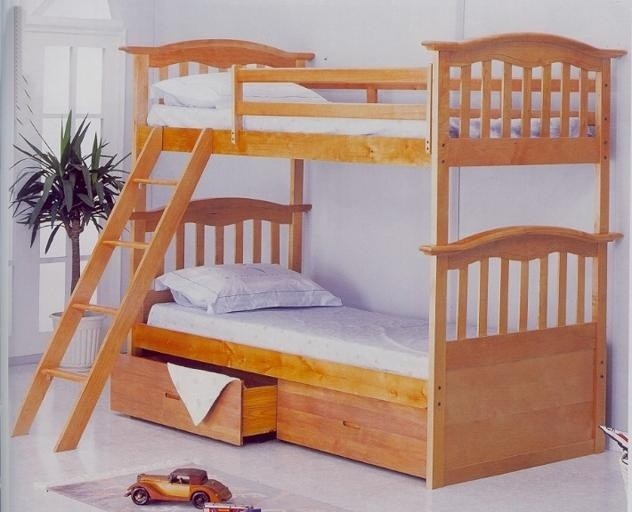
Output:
[8,109,131,372]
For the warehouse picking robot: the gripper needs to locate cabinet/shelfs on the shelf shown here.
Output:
[108,348,426,480]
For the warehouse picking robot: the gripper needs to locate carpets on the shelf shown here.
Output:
[45,461,352,512]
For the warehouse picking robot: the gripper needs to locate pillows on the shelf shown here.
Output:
[148,261,344,316]
[151,71,327,109]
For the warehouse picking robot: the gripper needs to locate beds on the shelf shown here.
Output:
[9,30,628,490]
